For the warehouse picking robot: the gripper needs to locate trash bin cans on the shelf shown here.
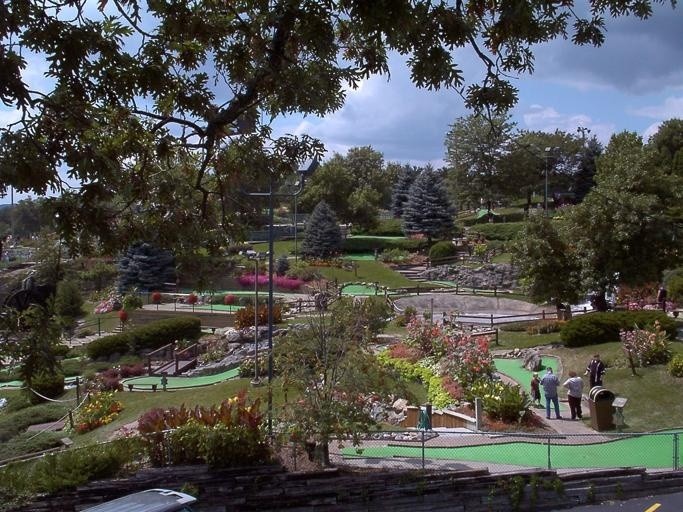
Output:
[419,404,432,429]
[588,385,615,431]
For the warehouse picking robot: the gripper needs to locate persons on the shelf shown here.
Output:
[586,354,604,389]
[540,367,562,419]
[530,372,541,404]
[563,370,585,419]
[657,284,666,306]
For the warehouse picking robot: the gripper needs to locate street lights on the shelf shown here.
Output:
[239,248,274,387]
[235,153,321,442]
[577,127,592,147]
[537,145,561,214]
